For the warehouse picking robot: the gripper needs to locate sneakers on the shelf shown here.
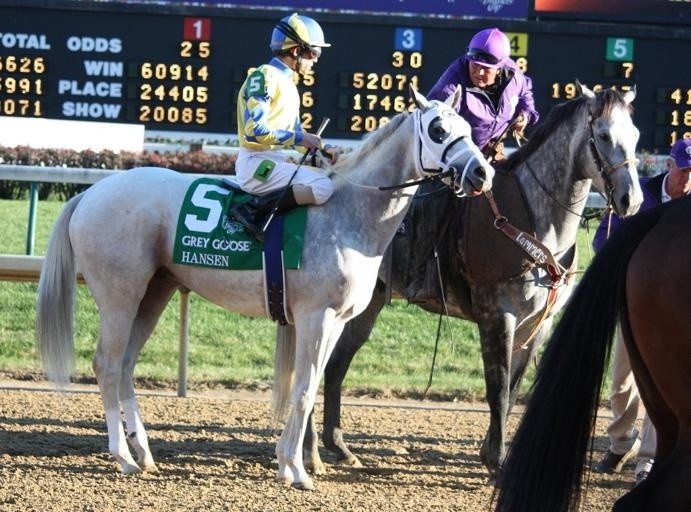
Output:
[597,439,641,474]
[635,455,654,482]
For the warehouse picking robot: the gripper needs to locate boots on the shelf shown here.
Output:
[415,259,446,301]
[230,185,297,239]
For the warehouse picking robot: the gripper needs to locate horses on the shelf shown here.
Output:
[36,82,495,490]
[301,78,644,478]
[496,194,691,512]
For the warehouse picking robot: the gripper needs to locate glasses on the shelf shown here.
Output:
[467,48,498,65]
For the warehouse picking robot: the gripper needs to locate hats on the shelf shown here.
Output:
[670,139,691,168]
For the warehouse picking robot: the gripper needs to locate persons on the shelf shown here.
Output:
[426,27,540,168]
[228,12,340,242]
[592,140,691,489]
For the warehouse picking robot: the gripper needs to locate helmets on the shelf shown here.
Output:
[465,28,511,68]
[270,12,331,49]
